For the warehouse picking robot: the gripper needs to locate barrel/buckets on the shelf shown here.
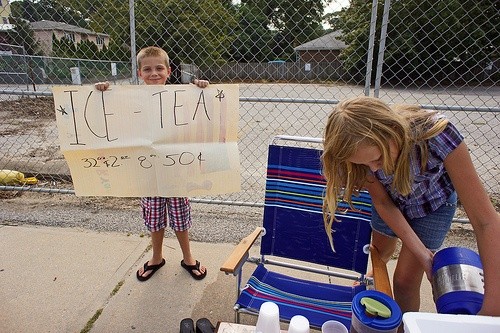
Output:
[431,247,485,315]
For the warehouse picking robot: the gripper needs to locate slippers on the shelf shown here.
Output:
[196,318,215,333]
[179,318,194,333]
[181,258,206,280]
[135,257,166,281]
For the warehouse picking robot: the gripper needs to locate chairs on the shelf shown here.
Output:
[220,134,374,333]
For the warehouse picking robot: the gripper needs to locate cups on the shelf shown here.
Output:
[288,315,309,333]
[256,302,280,333]
[322,321,348,333]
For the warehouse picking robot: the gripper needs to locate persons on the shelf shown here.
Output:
[95,46,209,280]
[322,97,500,317]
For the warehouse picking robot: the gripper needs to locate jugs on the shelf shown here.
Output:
[351,290,402,333]
